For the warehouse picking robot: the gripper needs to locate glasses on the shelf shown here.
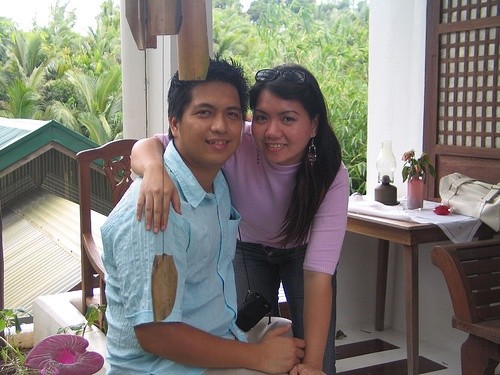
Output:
[255,69,310,86]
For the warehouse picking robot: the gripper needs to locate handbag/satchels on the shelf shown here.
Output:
[439,173,500,232]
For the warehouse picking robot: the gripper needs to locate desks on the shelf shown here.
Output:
[346,195,500,375]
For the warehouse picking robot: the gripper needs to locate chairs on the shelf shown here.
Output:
[73,137,139,334]
[430,238,500,375]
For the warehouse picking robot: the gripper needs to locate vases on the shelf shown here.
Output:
[407,176,425,210]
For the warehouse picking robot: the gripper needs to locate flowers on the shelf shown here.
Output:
[401,148,437,185]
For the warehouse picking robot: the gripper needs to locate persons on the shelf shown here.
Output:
[100,52,305,375]
[131,63,350,375]
